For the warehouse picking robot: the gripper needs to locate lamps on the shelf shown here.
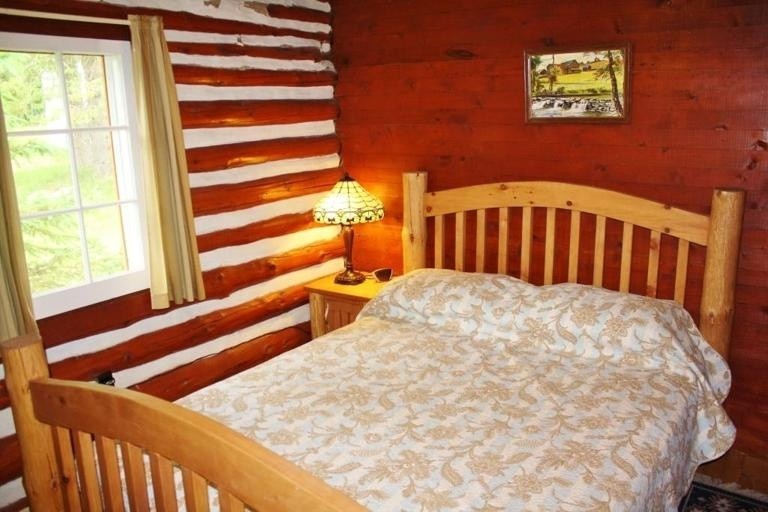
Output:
[311,169,388,288]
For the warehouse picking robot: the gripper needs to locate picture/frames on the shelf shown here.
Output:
[519,37,636,128]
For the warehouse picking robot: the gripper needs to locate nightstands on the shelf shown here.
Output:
[301,268,394,341]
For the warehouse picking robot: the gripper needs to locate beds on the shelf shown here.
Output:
[0,166,749,510]
